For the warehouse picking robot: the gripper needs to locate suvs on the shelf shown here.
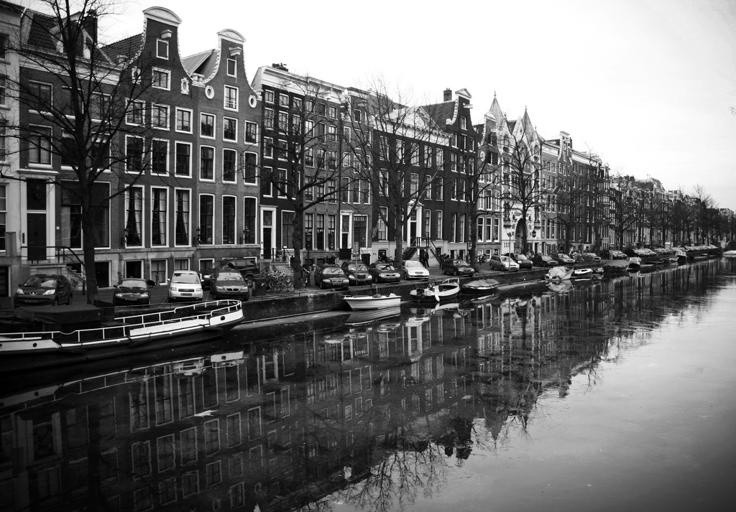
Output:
[210,267,249,301]
[199,259,258,289]
[166,269,205,302]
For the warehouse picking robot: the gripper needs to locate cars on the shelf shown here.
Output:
[14,273,73,307]
[304,259,431,287]
[488,243,718,271]
[112,278,152,306]
[442,259,475,277]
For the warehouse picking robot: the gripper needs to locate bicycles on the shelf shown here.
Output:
[261,270,294,293]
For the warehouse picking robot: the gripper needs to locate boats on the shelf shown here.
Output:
[0,298,245,378]
[345,308,402,326]
[343,293,400,309]
[407,298,460,317]
[1,335,247,412]
[462,279,500,294]
[408,278,460,305]
[544,250,736,292]
[462,291,501,306]
